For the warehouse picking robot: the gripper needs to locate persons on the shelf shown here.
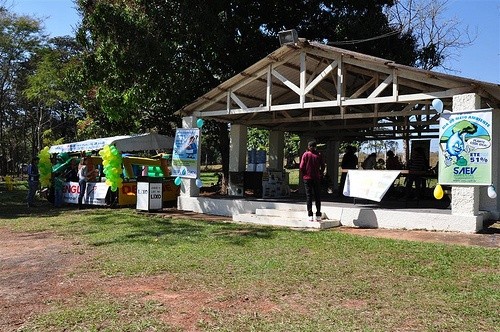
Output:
[298,140,322,221]
[319,145,426,192]
[186,136,197,158]
[26,158,38,207]
[76,157,91,210]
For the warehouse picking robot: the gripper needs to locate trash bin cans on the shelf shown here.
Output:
[136,176,164,213]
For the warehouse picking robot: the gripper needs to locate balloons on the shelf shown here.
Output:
[434,184,444,199]
[99,144,124,192]
[196,179,202,187]
[174,177,182,185]
[197,118,203,128]
[432,99,443,113]
[36,146,53,187]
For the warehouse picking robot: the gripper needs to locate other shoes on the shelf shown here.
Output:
[399,198,408,202]
[78,206,86,210]
[316,215,322,222]
[307,215,313,222]
[27,204,36,209]
[410,196,420,201]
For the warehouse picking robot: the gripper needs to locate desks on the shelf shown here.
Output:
[340,170,426,205]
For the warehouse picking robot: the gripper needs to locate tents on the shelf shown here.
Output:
[49,132,174,156]
